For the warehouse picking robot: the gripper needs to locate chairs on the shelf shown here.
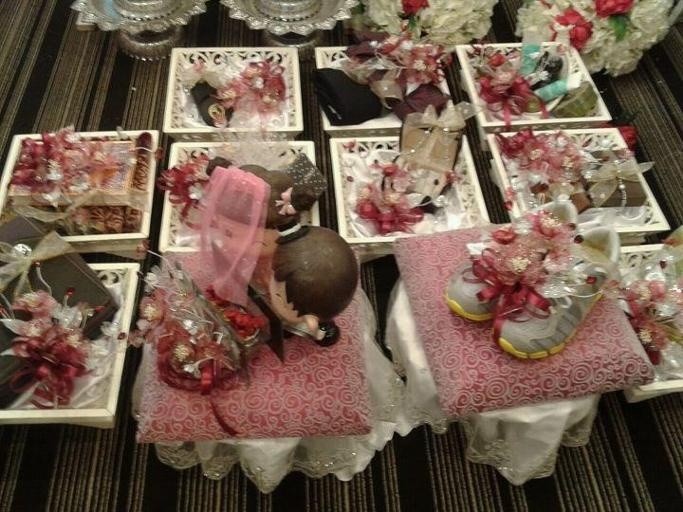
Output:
[446,199,621,361]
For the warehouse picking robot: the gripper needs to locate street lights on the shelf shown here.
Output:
[0,214,120,409]
